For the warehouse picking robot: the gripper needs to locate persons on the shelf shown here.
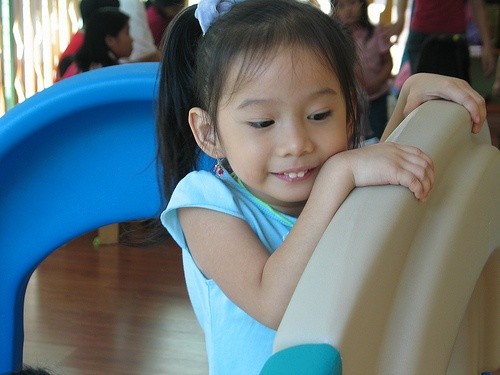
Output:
[155,0,486,375]
[329,0,500,141]
[54,0,188,248]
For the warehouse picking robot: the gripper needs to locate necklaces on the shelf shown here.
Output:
[230,171,295,229]
